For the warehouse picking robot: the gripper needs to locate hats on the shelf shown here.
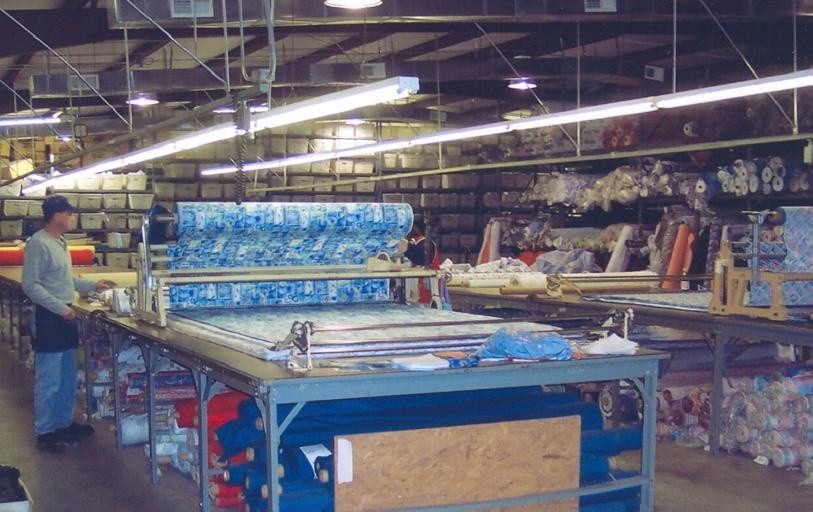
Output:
[41,195,84,215]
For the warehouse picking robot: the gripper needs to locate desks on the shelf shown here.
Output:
[0,266,672,512]
[447,287,813,456]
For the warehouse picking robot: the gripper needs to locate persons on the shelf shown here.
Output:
[20,196,116,452]
[398,214,438,310]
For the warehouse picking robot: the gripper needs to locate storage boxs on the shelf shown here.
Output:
[0,121,532,267]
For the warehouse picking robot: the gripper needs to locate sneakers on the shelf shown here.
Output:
[36,422,96,453]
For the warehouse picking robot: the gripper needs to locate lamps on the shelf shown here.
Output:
[508,78,537,89]
[20,76,419,194]
[200,68,813,176]
[249,98,267,112]
[211,103,238,113]
[125,92,158,104]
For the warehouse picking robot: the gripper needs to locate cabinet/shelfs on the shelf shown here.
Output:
[481,133,813,275]
